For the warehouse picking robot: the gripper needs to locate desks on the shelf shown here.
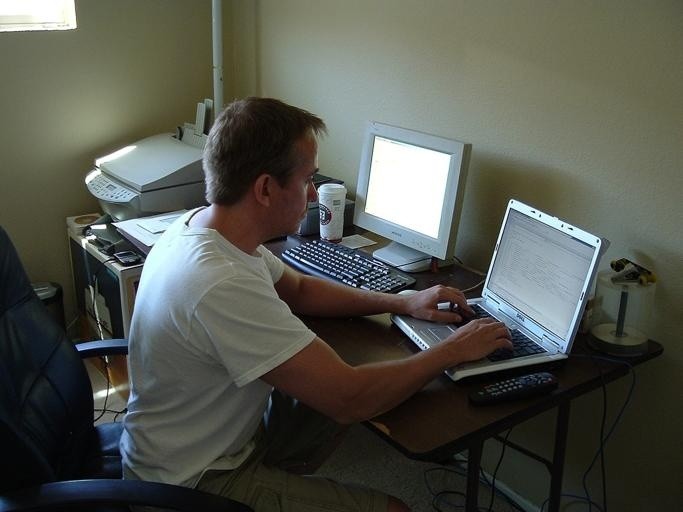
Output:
[111,194,669,511]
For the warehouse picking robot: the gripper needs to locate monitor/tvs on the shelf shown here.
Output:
[353,120,472,272]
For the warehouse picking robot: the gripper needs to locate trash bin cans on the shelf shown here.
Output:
[30,280,67,336]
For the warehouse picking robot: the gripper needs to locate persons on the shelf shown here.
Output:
[118,98,515,511]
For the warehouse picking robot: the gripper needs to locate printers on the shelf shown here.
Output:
[84,99,214,224]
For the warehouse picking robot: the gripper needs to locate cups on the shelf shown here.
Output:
[317,183,347,245]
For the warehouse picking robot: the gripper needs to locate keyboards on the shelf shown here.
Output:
[282,238,417,294]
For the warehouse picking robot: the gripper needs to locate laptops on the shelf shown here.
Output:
[389,199,603,382]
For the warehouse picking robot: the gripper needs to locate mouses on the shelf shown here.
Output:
[396,289,419,296]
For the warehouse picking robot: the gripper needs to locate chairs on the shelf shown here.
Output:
[1,226,262,512]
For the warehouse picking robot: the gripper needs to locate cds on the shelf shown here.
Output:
[591,322,648,346]
[75,216,99,225]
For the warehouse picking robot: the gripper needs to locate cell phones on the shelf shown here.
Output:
[113,250,141,266]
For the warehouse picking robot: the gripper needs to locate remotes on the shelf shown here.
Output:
[468,372,557,406]
